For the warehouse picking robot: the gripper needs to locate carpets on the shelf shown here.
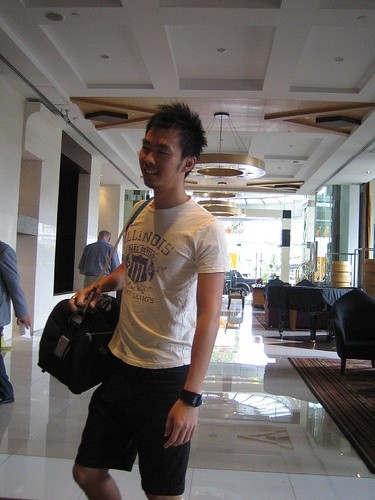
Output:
[288,355,375,475]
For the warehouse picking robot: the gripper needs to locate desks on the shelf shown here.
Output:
[265,285,364,349]
[250,283,265,310]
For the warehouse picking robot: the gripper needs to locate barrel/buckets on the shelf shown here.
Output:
[363,259,375,297]
[331,261,352,287]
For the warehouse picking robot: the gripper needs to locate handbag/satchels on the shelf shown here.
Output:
[37,293,120,394]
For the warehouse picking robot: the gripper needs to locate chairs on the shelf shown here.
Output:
[329,287,375,375]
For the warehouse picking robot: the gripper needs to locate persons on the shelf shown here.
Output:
[78,230,121,296]
[0,242,31,405]
[68,103,229,500]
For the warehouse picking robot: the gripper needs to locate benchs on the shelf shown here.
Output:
[227,283,251,310]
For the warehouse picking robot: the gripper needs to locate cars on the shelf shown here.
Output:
[222,270,263,294]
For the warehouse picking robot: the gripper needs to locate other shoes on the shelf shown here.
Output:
[0,398,14,404]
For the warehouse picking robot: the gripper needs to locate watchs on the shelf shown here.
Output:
[179,388,203,408]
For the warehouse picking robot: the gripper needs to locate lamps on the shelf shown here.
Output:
[185,111,266,219]
